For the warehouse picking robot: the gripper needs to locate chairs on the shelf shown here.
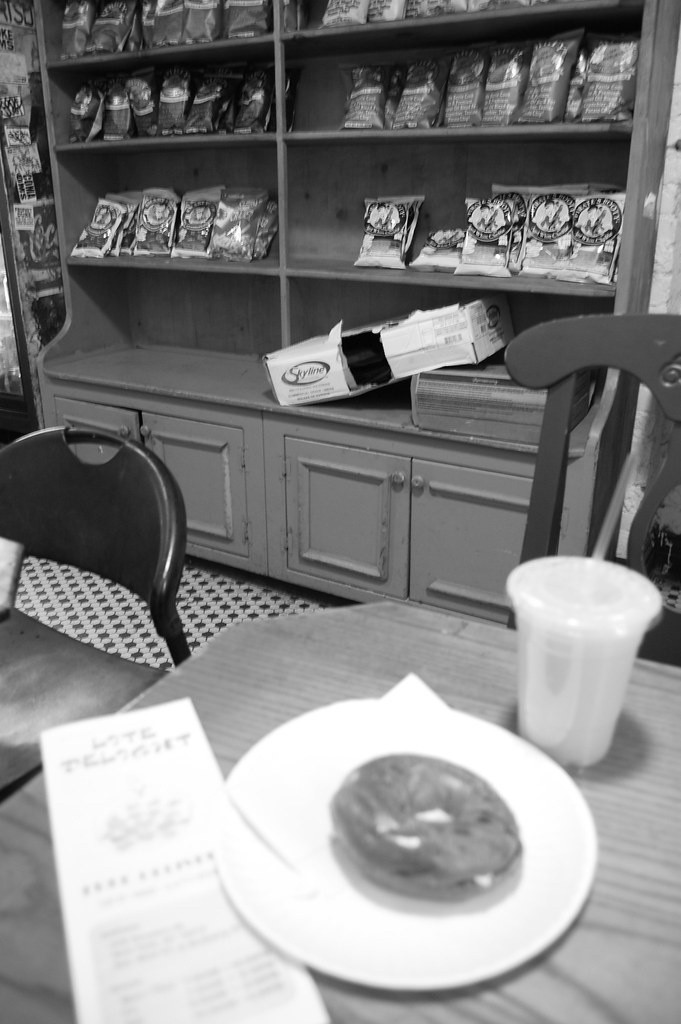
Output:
[1,420,190,669]
[502,313,680,670]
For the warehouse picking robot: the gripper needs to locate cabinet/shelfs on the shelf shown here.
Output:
[28,0,681,626]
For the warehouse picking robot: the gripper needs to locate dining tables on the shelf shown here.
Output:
[0,594,681,1024]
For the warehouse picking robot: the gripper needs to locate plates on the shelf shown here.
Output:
[207,698,599,991]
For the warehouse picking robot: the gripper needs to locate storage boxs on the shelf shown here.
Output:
[264,294,517,406]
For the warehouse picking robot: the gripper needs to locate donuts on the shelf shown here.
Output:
[330,753,519,893]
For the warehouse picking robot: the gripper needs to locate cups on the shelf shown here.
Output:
[506,555,663,767]
